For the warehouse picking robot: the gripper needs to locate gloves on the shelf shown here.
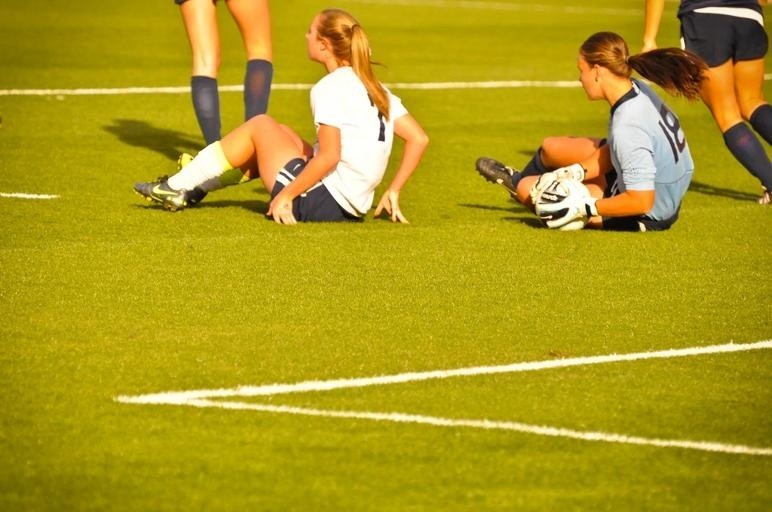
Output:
[531,163,599,229]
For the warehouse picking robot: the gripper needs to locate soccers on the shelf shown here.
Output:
[535,179,591,231]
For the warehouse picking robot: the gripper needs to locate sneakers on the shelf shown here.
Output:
[132,152,207,211]
[475,158,521,198]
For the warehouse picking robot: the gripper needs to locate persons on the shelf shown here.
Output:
[134,9,429,230]
[642,0,772,205]
[476,32,710,232]
[176,1,276,183]
